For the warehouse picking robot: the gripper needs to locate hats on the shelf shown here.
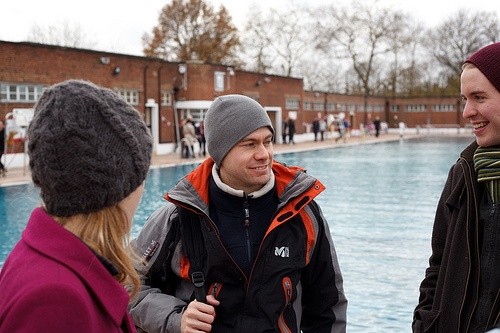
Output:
[28,80,154,216]
[203,95,275,169]
[463,41,500,95]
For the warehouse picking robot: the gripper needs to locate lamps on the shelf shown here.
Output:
[113,65,121,75]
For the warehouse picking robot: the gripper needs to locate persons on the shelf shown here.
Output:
[126,94,348,333]
[412,42,500,333]
[335,119,348,143]
[374,116,381,137]
[283,118,295,144]
[312,117,326,142]
[0,80,153,333]
[180,119,205,158]
[0,119,5,173]
